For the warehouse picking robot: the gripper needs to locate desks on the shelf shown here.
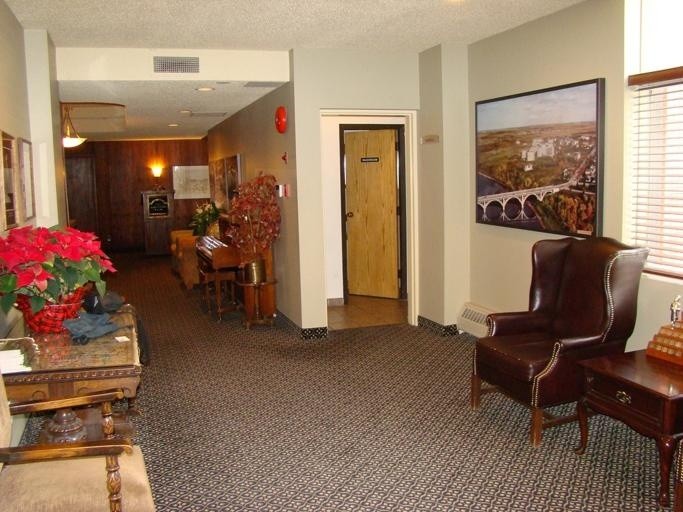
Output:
[0,301,145,442]
[234,277,277,331]
[576,350,683,508]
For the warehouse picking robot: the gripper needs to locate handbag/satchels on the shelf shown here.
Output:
[85,291,149,366]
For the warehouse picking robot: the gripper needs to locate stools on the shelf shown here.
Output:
[199,268,237,318]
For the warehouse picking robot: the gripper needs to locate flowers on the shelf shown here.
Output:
[183,197,226,237]
[226,171,281,259]
[0,224,117,313]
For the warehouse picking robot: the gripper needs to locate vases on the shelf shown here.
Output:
[238,258,266,283]
[17,285,90,337]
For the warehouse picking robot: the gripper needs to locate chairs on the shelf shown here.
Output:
[0,373,158,512]
[471,236,649,449]
[672,436,683,511]
[169,229,201,289]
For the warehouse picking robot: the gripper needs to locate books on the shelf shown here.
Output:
[0,337,37,373]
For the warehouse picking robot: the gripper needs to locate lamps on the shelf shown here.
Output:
[151,166,162,178]
[61,104,88,148]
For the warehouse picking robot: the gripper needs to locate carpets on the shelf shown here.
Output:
[153,255,177,267]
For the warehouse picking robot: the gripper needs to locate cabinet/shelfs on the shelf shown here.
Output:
[141,189,175,256]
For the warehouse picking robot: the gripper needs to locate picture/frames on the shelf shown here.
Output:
[1,130,37,232]
[474,78,604,237]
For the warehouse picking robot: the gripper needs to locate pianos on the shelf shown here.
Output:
[193,213,249,323]
